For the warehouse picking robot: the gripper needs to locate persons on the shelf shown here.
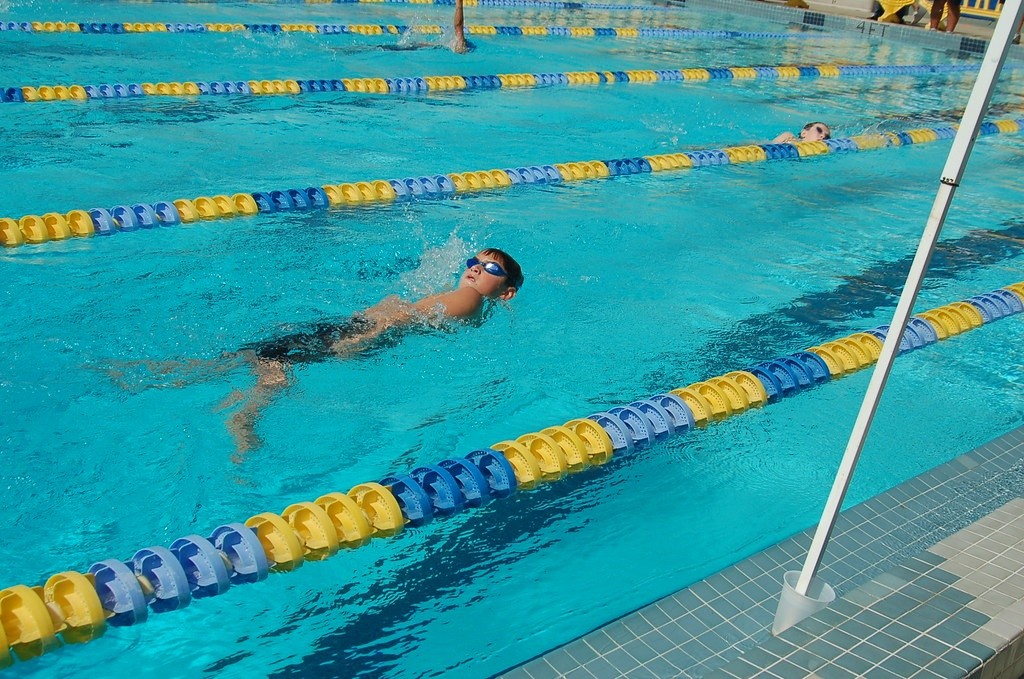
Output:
[871,0,962,34]
[90,248,525,467]
[676,121,831,150]
[1012,16,1024,43]
[326,0,476,55]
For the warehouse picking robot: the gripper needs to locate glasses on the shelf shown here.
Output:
[467,258,505,276]
[816,126,831,140]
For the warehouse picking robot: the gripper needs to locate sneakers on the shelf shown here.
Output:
[911,4,927,25]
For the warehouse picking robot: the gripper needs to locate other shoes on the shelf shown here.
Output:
[1012,33,1021,44]
[867,15,878,21]
